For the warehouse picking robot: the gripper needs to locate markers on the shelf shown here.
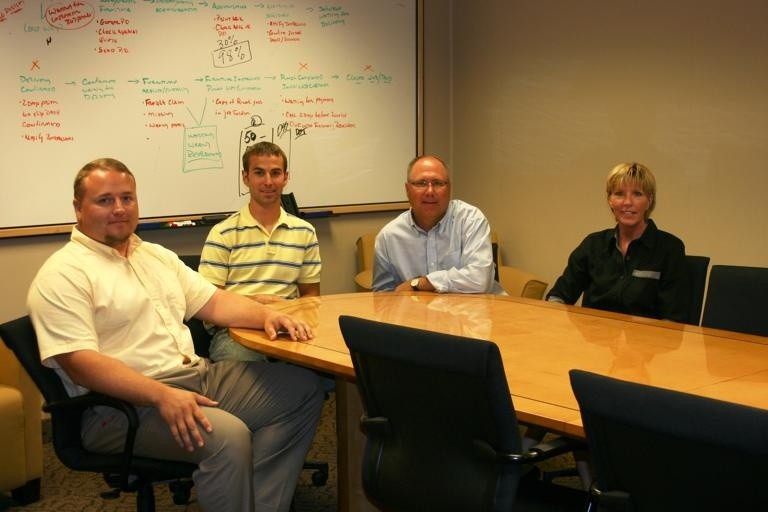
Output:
[300,211,333,217]
[165,221,197,227]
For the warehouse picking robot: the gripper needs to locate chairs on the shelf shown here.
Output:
[700,265,768,338]
[569,370,768,512]
[1,315,198,512]
[339,316,576,512]
[544,257,711,489]
[179,256,335,487]
[353,231,547,299]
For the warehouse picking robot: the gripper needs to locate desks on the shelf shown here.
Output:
[228,290,768,438]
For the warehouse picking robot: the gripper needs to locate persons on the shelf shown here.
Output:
[197,142,322,362]
[372,155,509,295]
[522,163,691,491]
[26,158,325,512]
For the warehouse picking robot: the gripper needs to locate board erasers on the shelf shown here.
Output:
[202,215,230,226]
[138,222,167,231]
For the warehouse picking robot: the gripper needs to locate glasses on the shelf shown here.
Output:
[408,180,450,188]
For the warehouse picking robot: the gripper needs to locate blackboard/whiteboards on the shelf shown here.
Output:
[1,2,424,239]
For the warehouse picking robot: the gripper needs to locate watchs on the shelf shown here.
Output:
[410,276,422,291]
